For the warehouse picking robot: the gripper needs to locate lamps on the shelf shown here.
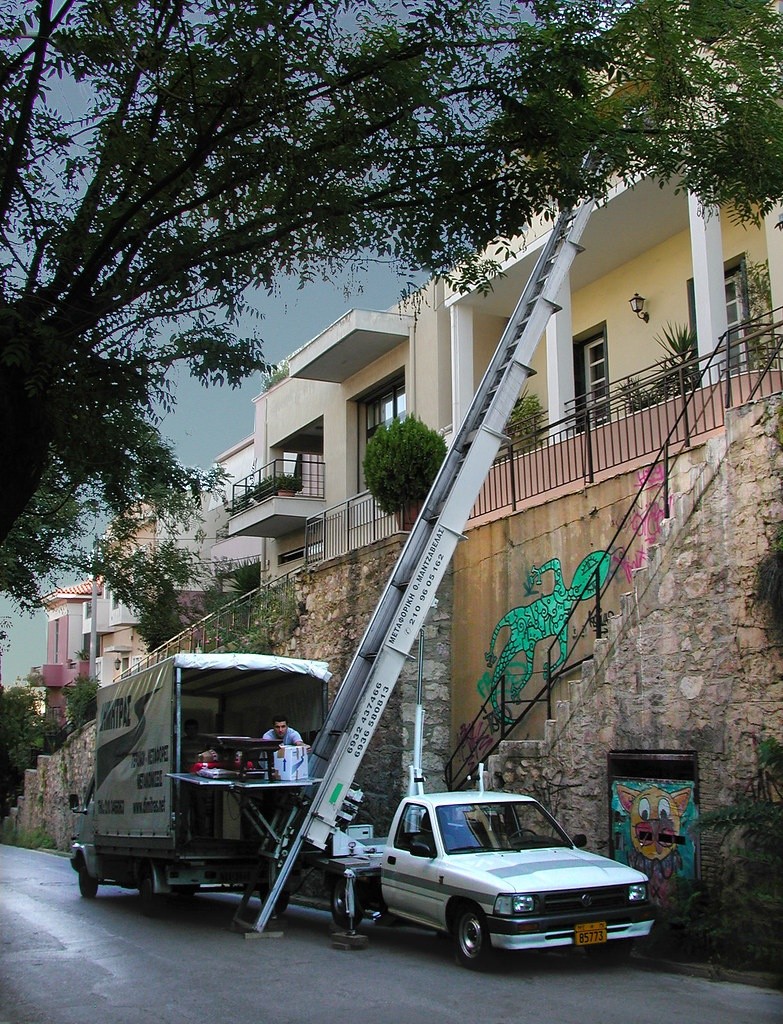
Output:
[627,292,648,323]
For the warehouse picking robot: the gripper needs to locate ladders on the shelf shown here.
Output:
[232,149,611,935]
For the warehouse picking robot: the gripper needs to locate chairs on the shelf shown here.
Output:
[474,814,512,848]
[410,812,457,850]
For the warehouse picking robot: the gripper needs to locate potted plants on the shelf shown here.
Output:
[229,475,304,514]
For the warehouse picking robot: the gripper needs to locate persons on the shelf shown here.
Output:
[262,715,313,754]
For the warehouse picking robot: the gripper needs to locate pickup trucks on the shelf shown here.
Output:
[320,791,662,973]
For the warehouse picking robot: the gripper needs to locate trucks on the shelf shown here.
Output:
[68,653,333,919]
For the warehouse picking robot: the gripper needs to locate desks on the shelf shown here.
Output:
[198,733,283,782]
